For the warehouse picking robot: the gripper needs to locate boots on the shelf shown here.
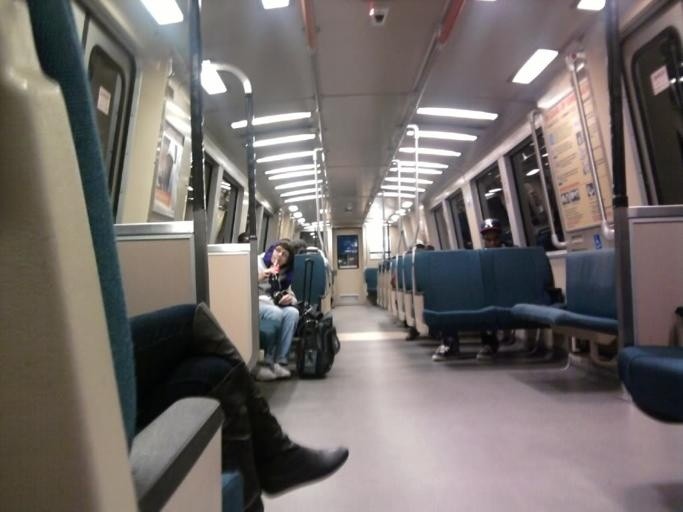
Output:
[192,301,349,497]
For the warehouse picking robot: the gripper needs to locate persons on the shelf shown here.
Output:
[237,232,308,257]
[403,323,420,340]
[433,330,500,359]
[256,238,300,382]
[480,217,509,251]
[128,303,347,511]
[406,240,436,252]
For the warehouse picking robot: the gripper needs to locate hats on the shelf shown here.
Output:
[479,218,501,234]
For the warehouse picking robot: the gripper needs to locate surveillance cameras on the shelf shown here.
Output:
[370,6,387,24]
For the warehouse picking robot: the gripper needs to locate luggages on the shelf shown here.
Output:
[295,258,341,378]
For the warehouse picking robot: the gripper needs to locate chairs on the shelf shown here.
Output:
[284,252,325,326]
[364,245,618,376]
[1,0,247,511]
[616,305,683,425]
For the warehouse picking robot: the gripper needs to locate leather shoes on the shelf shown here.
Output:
[405,328,420,341]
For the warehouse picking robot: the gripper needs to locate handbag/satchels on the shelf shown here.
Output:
[290,300,323,320]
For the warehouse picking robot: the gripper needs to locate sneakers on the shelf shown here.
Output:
[255,364,277,381]
[431,342,459,361]
[272,362,291,378]
[476,343,498,360]
